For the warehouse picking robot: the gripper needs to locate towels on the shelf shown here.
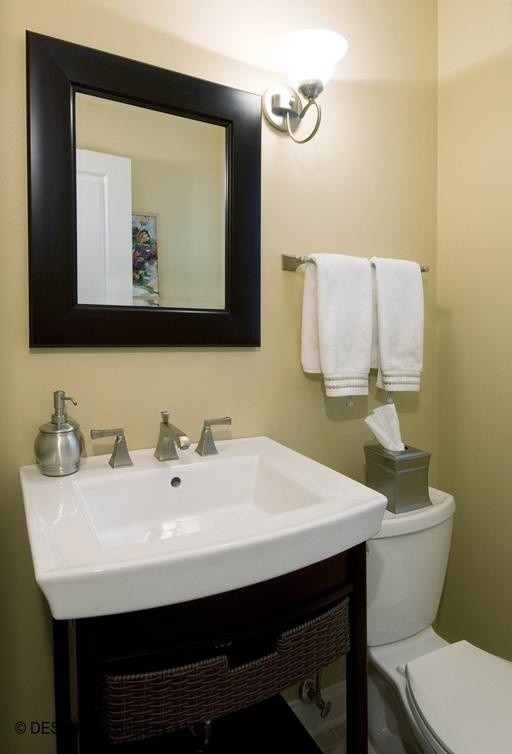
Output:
[301,252,373,400]
[369,254,424,394]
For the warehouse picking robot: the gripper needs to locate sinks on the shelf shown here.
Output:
[18,435,389,621]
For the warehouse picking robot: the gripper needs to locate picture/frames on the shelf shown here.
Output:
[131,211,162,307]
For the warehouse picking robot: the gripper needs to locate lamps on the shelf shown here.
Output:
[260,26,350,144]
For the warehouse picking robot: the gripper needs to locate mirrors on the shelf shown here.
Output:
[25,29,265,350]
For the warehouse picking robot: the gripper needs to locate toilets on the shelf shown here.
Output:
[365,486,512,754]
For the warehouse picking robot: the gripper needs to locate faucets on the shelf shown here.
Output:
[152,409,190,463]
